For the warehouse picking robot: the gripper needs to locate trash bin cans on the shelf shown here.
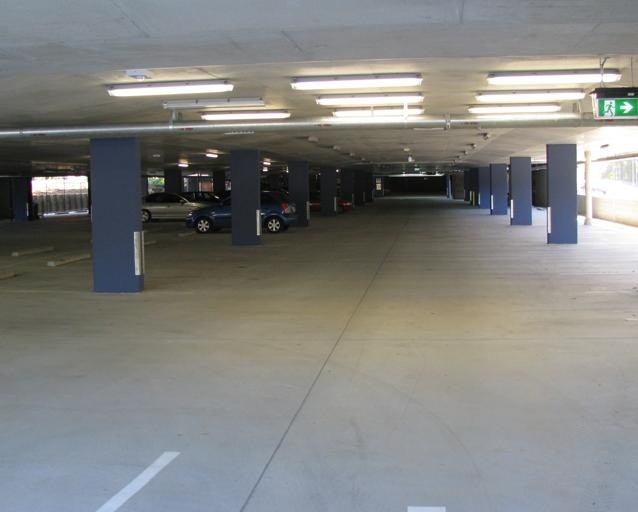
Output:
[28,202,39,220]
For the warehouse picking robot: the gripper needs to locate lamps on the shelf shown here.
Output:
[290,72,423,91]
[106,79,235,98]
[467,104,563,113]
[316,90,425,108]
[160,96,267,111]
[200,108,292,123]
[474,87,587,105]
[485,69,622,86]
[333,108,425,118]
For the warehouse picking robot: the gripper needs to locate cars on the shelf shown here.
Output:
[140,182,356,234]
